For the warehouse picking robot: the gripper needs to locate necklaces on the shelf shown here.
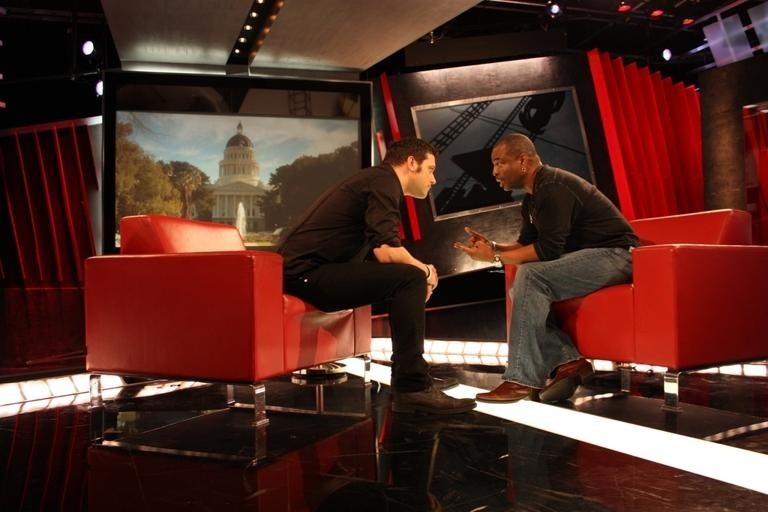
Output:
[528,201,537,224]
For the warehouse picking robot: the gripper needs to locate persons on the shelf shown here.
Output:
[319,400,578,512]
[453,134,640,403]
[277,137,477,416]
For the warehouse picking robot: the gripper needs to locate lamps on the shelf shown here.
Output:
[545,1,566,19]
[81,39,97,58]
[96,80,104,97]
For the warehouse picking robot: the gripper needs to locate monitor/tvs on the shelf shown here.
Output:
[101,68,372,256]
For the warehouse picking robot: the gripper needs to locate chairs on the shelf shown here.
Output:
[498,207,766,414]
[85,398,373,511]
[81,213,374,471]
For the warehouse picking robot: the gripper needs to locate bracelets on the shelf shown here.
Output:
[425,264,433,282]
[490,241,498,252]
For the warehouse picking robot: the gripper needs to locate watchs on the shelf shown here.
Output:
[492,254,502,264]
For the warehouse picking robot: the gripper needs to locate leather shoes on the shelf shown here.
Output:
[392,376,477,414]
[476,380,543,401]
[538,357,595,401]
